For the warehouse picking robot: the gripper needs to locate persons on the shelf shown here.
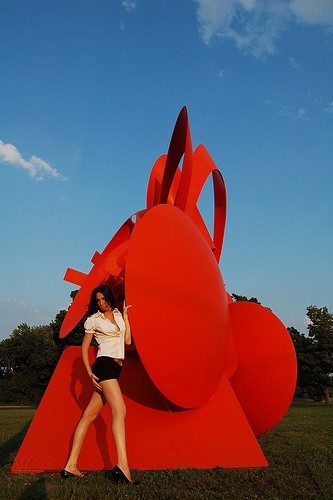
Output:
[63,285,141,486]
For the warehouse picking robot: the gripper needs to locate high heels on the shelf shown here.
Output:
[61,468,89,482]
[112,466,141,488]
